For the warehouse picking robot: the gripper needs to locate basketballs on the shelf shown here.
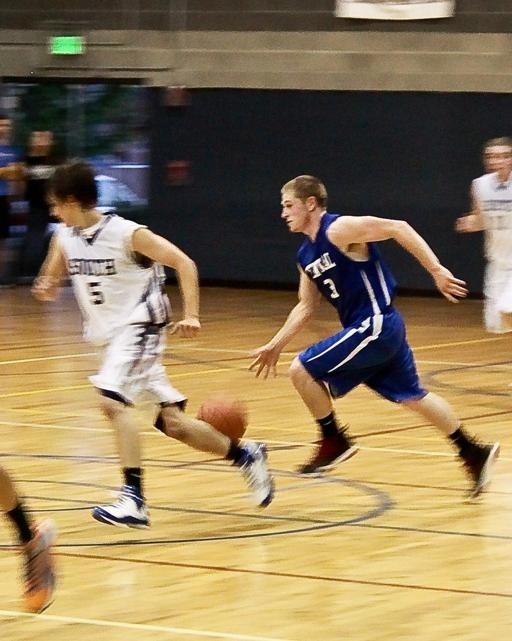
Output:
[198,402,248,441]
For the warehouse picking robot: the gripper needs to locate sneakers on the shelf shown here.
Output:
[231,441,273,508]
[302,434,359,474]
[92,486,150,530]
[26,519,57,614]
[460,444,500,496]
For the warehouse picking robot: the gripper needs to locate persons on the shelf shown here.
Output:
[245,175,500,506]
[455,137,512,337]
[0,130,71,286]
[30,159,278,532]
[0,466,60,616]
[1,115,28,289]
